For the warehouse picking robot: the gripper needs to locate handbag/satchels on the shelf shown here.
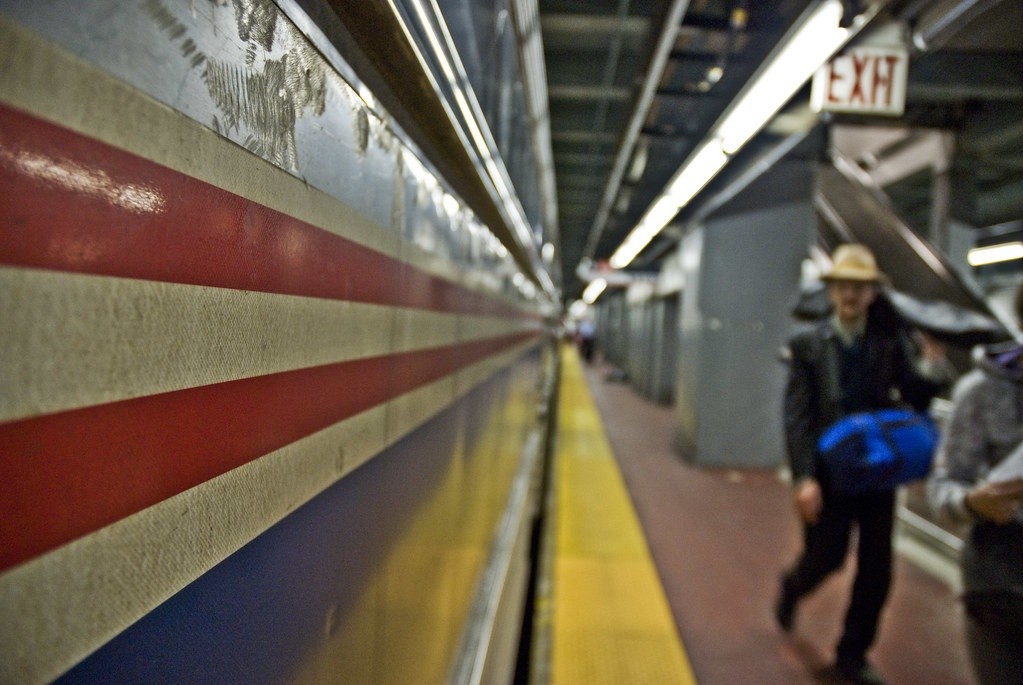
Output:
[819,408,939,486]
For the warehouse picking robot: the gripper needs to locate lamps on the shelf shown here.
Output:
[609,0,883,269]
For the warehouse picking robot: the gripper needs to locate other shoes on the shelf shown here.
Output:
[836,654,886,685]
[775,569,795,629]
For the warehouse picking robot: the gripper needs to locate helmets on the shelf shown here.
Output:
[819,245,882,283]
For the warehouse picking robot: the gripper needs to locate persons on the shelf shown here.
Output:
[579,316,595,365]
[771,243,934,685]
[928,283,1023,685]
